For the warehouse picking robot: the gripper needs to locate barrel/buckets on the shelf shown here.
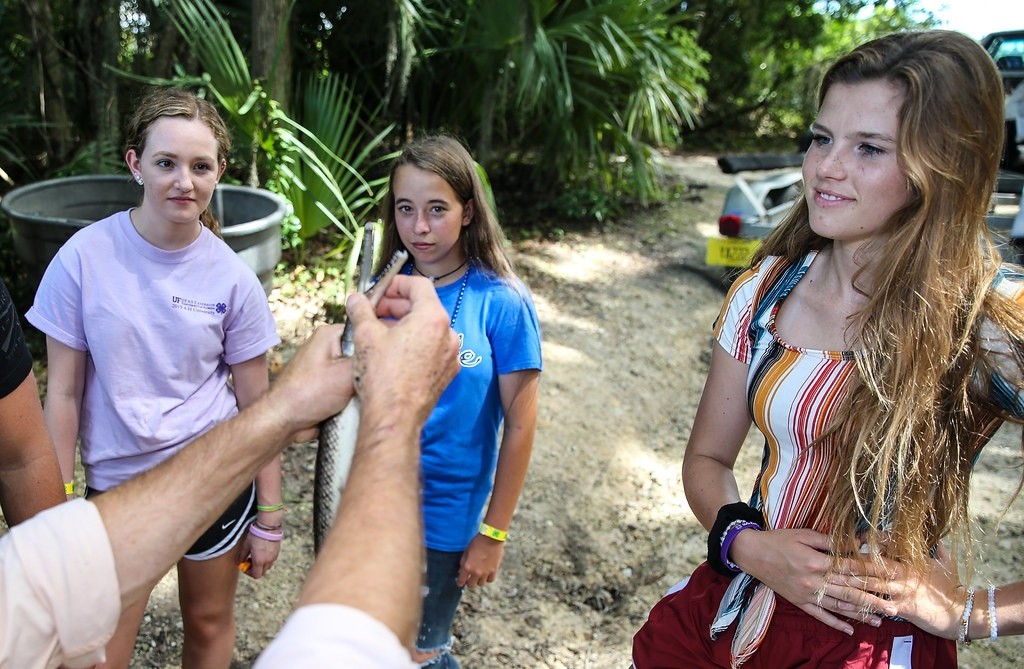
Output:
[1,173,287,303]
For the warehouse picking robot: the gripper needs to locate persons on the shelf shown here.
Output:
[0,272,463,669]
[21,88,285,669]
[368,132,544,668]
[628,28,1024,668]
[0,277,67,528]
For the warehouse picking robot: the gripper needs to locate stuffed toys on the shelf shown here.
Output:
[707,501,766,578]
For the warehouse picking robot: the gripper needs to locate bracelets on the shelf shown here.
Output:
[255,520,283,530]
[479,522,506,542]
[63,480,75,495]
[987,584,999,641]
[250,523,283,542]
[958,587,974,646]
[257,503,285,512]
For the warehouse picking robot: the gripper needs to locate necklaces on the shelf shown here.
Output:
[406,256,472,327]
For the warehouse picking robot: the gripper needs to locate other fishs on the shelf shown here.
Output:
[314,316,361,557]
